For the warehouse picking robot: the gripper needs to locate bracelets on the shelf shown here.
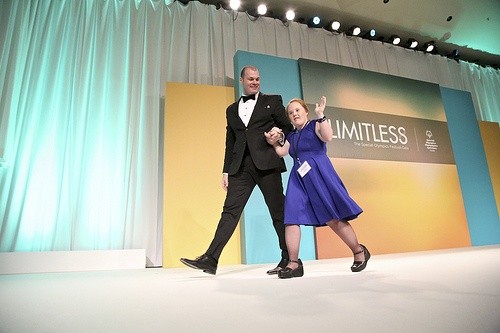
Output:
[318,115,326,123]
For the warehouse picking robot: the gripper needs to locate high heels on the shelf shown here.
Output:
[350,244,371,272]
[278,259,304,278]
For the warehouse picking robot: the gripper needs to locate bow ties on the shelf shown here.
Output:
[241,94,255,103]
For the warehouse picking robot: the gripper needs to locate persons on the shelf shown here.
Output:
[264,96,371,279]
[180,67,292,274]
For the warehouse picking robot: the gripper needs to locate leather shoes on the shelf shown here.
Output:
[179,253,218,275]
[267,266,283,275]
